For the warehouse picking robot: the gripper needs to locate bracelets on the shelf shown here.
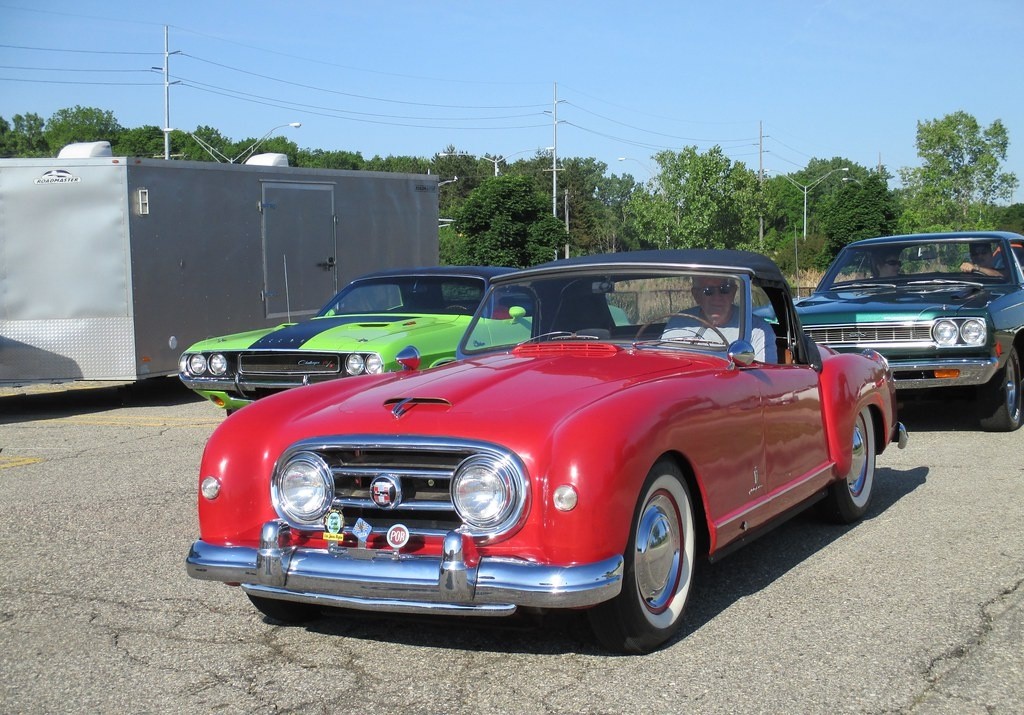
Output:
[974,264,980,270]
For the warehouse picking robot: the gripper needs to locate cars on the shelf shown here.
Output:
[993,242,1024,270]
[176,263,631,417]
[767,230,1024,432]
[180,248,910,658]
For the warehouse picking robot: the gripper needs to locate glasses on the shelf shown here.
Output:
[695,284,738,296]
[883,260,902,267]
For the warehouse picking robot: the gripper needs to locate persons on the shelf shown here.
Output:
[959,243,1008,278]
[874,249,901,278]
[478,286,513,320]
[660,276,777,365]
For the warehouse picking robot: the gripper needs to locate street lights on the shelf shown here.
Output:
[439,146,556,179]
[163,121,301,165]
[763,167,850,245]
